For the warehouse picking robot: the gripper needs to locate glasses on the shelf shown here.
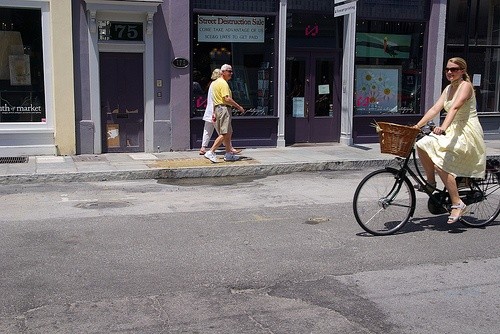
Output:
[226,70,233,73]
[444,68,464,73]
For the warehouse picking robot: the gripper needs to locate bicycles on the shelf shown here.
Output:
[353,120,500,236]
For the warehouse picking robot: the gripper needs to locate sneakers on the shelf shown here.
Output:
[204,151,219,163]
[224,154,239,161]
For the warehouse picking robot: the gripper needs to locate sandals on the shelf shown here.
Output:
[414,181,436,193]
[447,201,468,224]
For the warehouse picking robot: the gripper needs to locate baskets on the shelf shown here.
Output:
[376,122,420,156]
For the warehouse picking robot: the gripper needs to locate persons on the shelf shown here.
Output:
[199,68,242,154]
[410,57,486,223]
[204,64,245,162]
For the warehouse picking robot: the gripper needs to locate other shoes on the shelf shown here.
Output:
[233,149,241,153]
[199,150,204,155]
